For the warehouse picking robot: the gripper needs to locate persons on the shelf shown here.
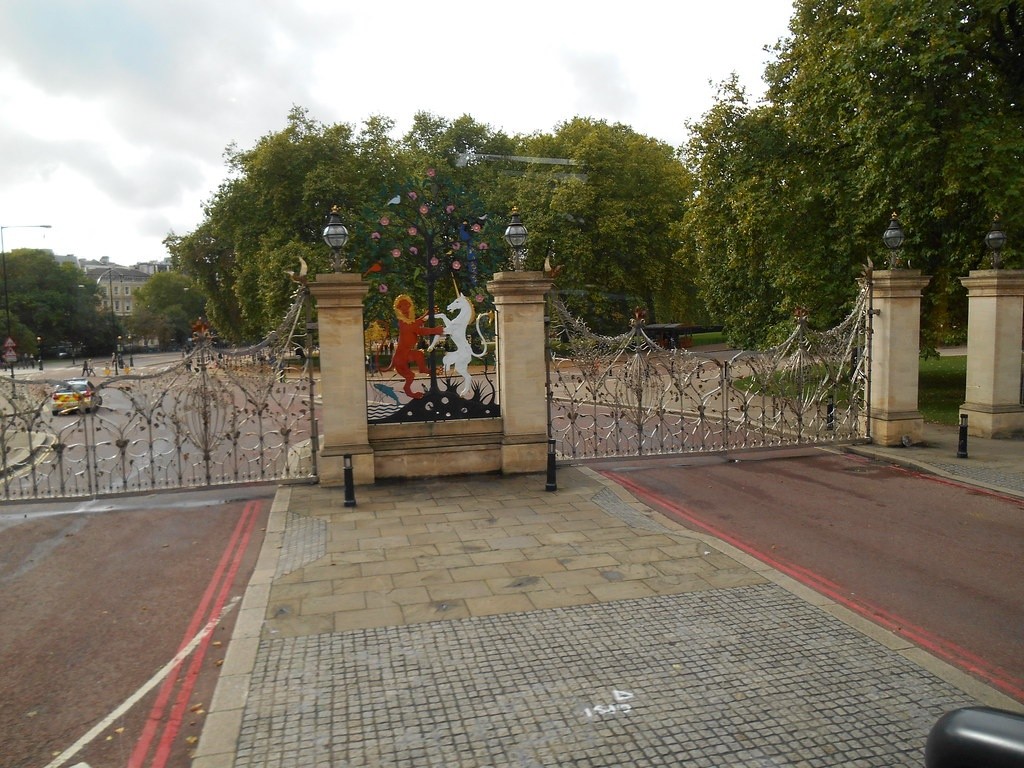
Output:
[366,352,375,376]
[2,354,36,370]
[81,352,222,378]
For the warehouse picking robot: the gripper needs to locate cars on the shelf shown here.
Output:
[51,377,103,416]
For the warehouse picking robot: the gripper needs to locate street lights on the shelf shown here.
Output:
[1,224,52,400]
